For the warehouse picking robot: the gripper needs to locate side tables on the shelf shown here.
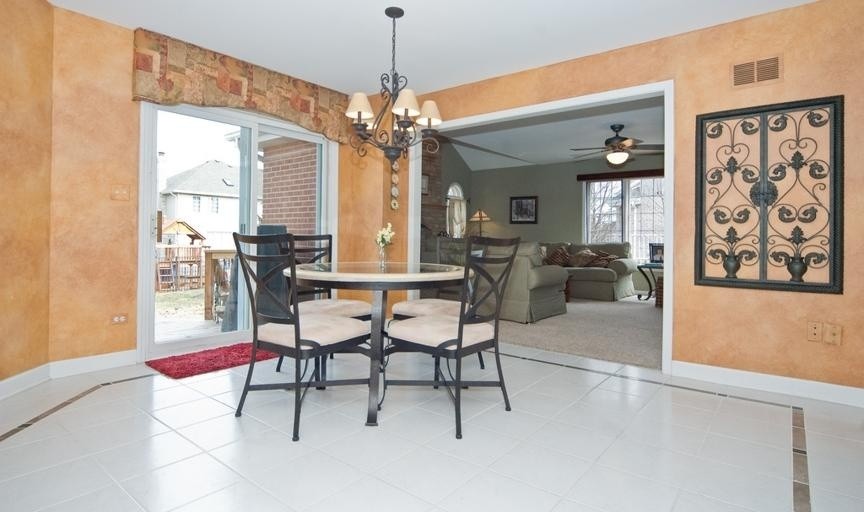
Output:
[638,264,663,301]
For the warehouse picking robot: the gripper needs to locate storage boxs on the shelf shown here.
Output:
[655,277,663,307]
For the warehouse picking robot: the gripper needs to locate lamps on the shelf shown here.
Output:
[345,7,441,166]
[606,149,629,166]
[468,209,491,236]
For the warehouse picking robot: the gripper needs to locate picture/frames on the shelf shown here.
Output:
[649,243,664,263]
[422,174,429,195]
[509,196,538,224]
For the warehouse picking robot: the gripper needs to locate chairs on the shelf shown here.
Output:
[371,235,523,439]
[256,225,335,390]
[391,233,491,390]
[232,232,387,442]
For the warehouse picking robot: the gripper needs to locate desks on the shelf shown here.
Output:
[205,250,237,324]
[281,261,477,424]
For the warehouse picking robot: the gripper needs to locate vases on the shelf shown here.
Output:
[378,243,385,259]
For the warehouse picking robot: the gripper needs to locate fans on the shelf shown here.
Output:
[570,124,663,159]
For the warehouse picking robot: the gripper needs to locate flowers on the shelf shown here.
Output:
[375,222,394,244]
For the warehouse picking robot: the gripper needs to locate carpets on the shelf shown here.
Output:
[479,301,662,369]
[144,341,281,379]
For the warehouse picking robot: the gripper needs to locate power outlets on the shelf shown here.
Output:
[808,322,821,342]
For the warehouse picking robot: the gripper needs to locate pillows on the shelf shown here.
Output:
[543,247,617,268]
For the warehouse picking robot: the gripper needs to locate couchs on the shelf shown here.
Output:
[542,242,639,302]
[475,242,569,325]
